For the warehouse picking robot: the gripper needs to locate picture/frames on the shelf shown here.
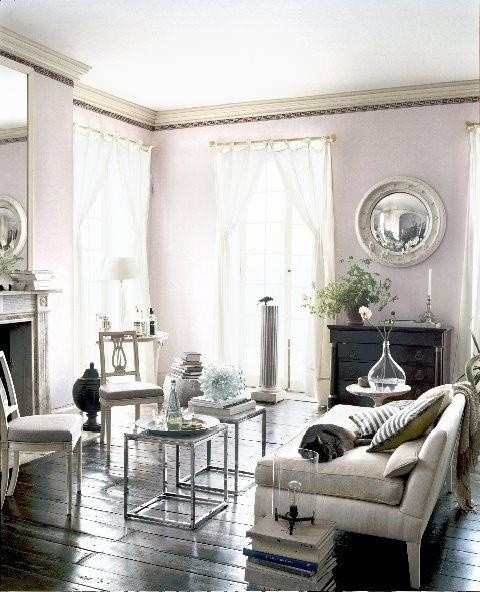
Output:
[124,422,230,529]
[345,383,413,410]
[175,403,268,503]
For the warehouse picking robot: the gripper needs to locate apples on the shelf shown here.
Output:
[427,269,433,296]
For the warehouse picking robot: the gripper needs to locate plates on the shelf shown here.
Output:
[327,323,453,412]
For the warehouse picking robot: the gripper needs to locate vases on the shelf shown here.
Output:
[359,305,373,323]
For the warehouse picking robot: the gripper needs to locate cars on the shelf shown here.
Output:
[150,409,165,423]
[358,374,371,388]
[180,406,195,420]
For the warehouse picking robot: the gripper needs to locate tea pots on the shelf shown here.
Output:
[355,176,447,269]
[0,195,29,264]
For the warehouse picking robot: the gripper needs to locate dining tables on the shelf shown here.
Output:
[302,254,396,326]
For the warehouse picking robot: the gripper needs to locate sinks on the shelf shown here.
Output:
[254,384,466,588]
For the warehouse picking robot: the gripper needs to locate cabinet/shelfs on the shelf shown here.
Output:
[132,305,156,340]
[166,379,182,430]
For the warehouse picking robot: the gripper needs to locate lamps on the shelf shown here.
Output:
[0,351,84,517]
[97,330,166,459]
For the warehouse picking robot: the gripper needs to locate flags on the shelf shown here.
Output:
[368,339,408,390]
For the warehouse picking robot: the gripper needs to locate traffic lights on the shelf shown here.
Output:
[384,438,426,479]
[360,378,453,454]
[349,397,417,438]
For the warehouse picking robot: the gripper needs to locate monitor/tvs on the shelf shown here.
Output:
[170,351,204,379]
[242,513,339,591]
[11,268,55,291]
